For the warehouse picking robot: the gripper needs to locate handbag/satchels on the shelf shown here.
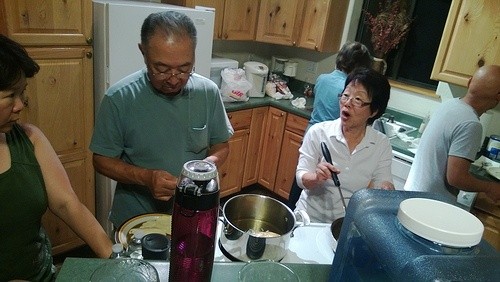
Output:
[220,68,251,103]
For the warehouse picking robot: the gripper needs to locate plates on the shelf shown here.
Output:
[115,212,171,250]
[486,165,500,180]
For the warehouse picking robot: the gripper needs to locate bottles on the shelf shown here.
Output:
[167,160,221,282]
[329,187,500,281]
[108,244,131,259]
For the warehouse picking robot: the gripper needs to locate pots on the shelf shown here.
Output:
[329,216,344,253]
[218,194,311,262]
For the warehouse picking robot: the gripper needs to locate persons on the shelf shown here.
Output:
[88,10,236,245]
[305,39,374,132]
[402,64,500,203]
[0,34,120,282]
[293,66,397,225]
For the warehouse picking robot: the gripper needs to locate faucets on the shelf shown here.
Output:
[382,113,395,123]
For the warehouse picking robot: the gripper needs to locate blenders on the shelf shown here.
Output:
[282,60,298,89]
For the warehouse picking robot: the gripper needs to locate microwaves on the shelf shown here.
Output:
[210,54,238,94]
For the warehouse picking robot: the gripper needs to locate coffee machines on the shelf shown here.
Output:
[269,55,288,76]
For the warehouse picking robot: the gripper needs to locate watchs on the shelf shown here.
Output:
[109,242,124,259]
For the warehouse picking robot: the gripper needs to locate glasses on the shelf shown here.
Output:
[146,58,197,79]
[338,92,372,110]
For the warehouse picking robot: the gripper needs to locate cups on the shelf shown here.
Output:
[141,233,169,260]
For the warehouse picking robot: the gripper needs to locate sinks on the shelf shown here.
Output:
[372,116,418,140]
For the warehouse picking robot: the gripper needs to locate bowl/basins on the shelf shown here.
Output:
[88,258,160,282]
[238,259,302,282]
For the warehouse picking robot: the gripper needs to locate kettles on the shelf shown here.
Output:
[243,61,268,98]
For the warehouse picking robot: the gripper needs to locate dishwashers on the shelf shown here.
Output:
[391,158,411,191]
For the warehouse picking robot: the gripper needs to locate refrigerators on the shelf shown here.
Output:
[92,0,216,234]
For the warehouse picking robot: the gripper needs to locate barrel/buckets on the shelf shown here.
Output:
[486,135,500,153]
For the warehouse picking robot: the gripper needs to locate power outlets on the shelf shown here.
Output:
[306,62,318,74]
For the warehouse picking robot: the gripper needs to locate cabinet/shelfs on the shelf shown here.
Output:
[243,107,267,188]
[211,108,251,197]
[0,0,96,258]
[159,0,350,52]
[430,0,499,89]
[275,113,309,200]
[258,106,288,191]
